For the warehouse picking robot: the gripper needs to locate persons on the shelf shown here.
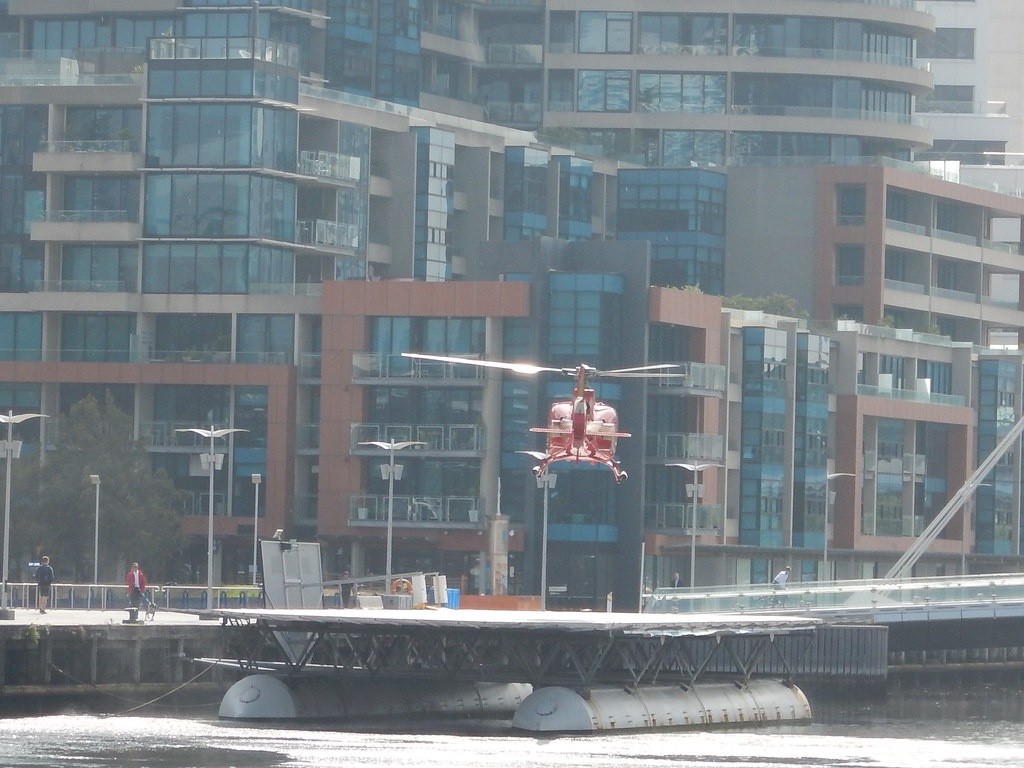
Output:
[340,568,376,598]
[772,565,793,605]
[125,562,146,618]
[34,555,55,614]
[669,573,683,612]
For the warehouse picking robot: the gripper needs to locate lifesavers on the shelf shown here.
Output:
[391,579,414,597]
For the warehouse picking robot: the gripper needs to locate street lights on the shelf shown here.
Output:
[663,459,727,588]
[958,469,994,577]
[252,473,262,587]
[514,450,554,612]
[823,458,857,583]
[175,424,250,614]
[0,409,50,622]
[358,439,429,598]
[90,474,101,601]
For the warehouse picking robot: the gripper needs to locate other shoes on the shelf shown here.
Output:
[39,608,47,614]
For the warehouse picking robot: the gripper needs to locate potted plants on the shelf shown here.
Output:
[721,293,950,348]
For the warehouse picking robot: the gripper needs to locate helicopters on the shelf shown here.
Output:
[403,347,687,481]
